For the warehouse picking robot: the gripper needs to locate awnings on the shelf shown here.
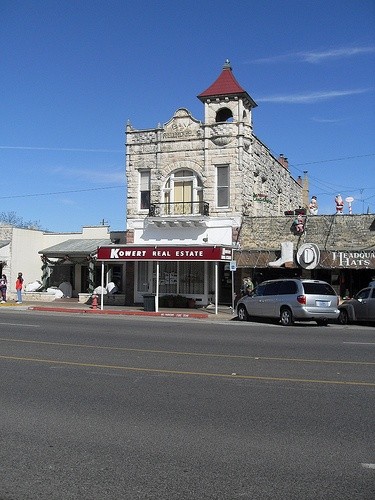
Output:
[97,243,234,315]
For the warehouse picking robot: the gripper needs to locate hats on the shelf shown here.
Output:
[296,243,320,270]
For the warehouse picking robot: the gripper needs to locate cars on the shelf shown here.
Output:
[338,286,375,325]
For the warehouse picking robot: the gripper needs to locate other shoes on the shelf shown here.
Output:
[17,302,22,303]
[1,301,5,304]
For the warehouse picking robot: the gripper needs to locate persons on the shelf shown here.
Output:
[295,214,304,232]
[334,193,344,214]
[242,276,253,292]
[0,274,7,304]
[16,272,24,304]
[308,196,318,215]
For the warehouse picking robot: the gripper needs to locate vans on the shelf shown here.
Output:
[236,278,341,326]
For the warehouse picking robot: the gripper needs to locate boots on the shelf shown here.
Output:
[269,242,293,268]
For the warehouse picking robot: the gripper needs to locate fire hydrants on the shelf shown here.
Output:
[91,290,99,310]
